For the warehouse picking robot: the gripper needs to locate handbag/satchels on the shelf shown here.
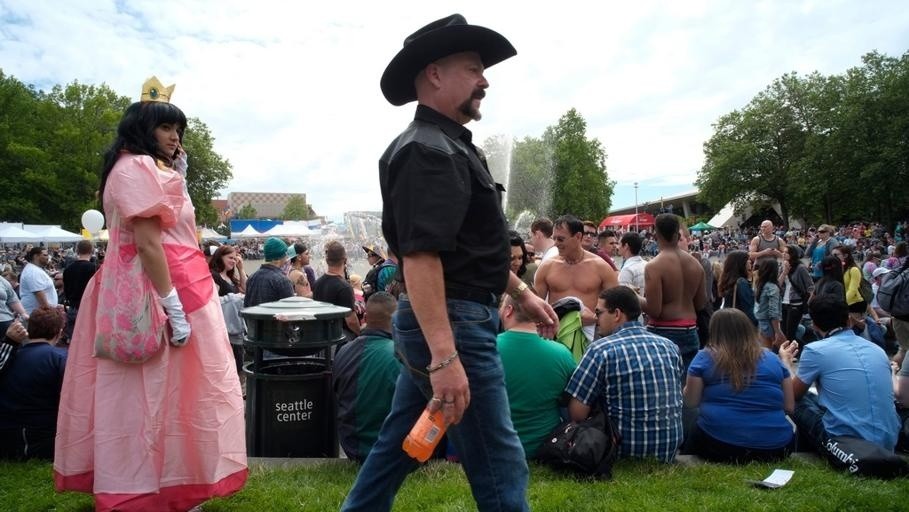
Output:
[826,438,908,479]
[538,417,621,477]
[877,266,909,323]
[859,279,874,303]
[93,252,167,363]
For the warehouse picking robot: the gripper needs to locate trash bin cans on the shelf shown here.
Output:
[238,292,353,458]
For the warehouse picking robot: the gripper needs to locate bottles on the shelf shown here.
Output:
[402,397,455,463]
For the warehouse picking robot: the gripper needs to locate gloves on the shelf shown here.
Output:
[156,284,191,349]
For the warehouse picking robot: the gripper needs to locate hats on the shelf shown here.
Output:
[380,14,516,108]
[872,267,890,278]
[786,244,804,258]
[363,244,385,259]
[286,242,306,260]
[264,237,287,261]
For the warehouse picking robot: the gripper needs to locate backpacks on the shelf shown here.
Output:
[362,262,395,301]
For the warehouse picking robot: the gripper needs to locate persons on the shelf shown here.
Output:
[53,76,249,512]
[0,213,909,464]
[338,15,560,512]
